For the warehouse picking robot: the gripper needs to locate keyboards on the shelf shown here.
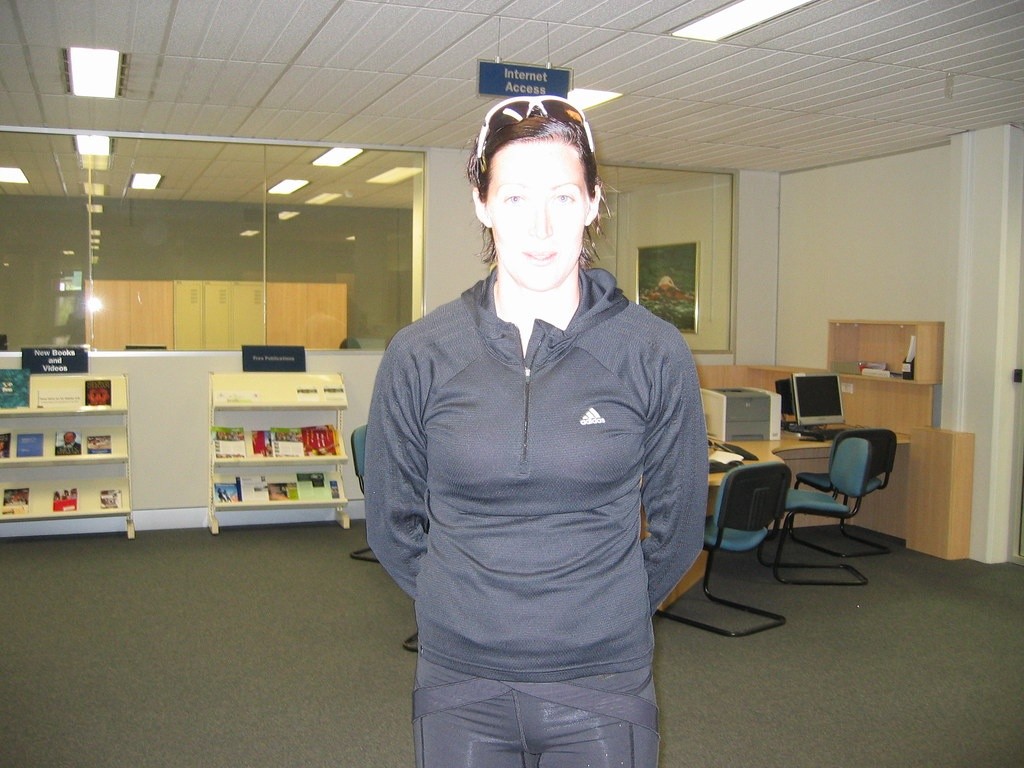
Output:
[803,429,845,439]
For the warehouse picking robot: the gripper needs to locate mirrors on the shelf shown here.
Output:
[0,130,426,353]
[585,161,740,354]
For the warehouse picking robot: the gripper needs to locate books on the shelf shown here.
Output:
[213,425,341,502]
[0,370,122,514]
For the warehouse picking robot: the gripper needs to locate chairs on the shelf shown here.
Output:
[655,462,792,637]
[789,428,897,558]
[757,438,873,586]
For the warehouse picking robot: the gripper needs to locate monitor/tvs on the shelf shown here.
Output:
[791,372,845,436]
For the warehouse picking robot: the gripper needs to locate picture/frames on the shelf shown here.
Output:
[636,240,701,335]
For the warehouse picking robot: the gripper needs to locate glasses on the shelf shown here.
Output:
[474,96,595,173]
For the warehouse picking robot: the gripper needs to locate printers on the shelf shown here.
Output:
[700,385,782,444]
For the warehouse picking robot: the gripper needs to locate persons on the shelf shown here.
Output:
[646,277,695,303]
[87,385,110,405]
[0,441,9,458]
[56,431,81,455]
[365,96,710,768]
[9,490,27,504]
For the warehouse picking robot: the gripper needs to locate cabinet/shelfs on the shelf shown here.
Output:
[266,282,347,349]
[608,318,975,613]
[0,369,136,540]
[85,280,173,351]
[174,280,262,350]
[208,370,350,535]
